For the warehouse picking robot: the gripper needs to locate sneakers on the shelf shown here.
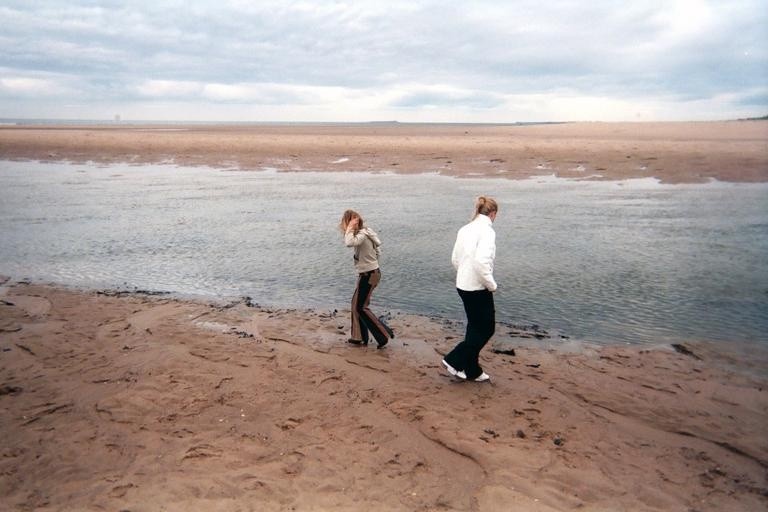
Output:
[475,371,490,382]
[441,359,468,380]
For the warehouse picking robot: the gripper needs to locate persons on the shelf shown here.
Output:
[441,195,499,382]
[340,210,395,349]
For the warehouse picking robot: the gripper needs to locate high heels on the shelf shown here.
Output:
[377,333,395,348]
[349,339,368,345]
